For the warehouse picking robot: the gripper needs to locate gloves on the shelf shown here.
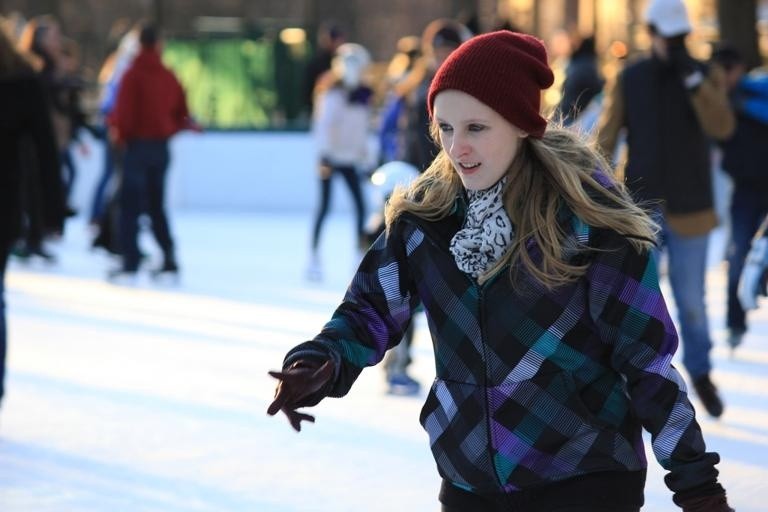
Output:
[266,358,335,432]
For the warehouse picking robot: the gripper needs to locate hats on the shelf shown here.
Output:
[427,30,554,138]
[646,0,690,38]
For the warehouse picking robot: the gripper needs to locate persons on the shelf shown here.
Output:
[11,12,191,283]
[269,1,768,419]
[0,0,54,408]
[264,30,737,511]
